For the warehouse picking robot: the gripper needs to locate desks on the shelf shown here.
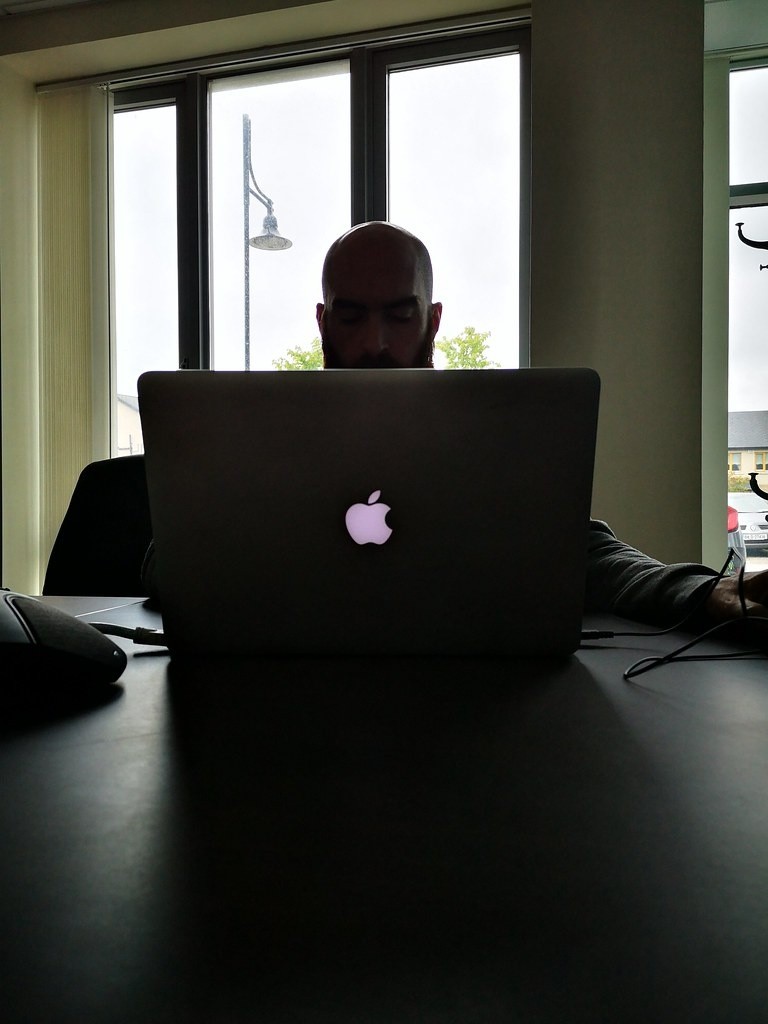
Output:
[0,595,768,1024]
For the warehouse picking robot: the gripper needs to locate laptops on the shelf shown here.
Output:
[136,367,601,659]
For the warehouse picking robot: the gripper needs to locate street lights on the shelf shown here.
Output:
[243,111,291,370]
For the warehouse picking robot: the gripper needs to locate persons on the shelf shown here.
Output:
[146,214,767,685]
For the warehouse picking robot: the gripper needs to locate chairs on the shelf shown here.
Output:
[44,452,153,595]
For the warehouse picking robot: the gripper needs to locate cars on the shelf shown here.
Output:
[727,490,768,550]
[724,506,746,582]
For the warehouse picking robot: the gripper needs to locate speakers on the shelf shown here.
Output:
[0,589,128,688]
